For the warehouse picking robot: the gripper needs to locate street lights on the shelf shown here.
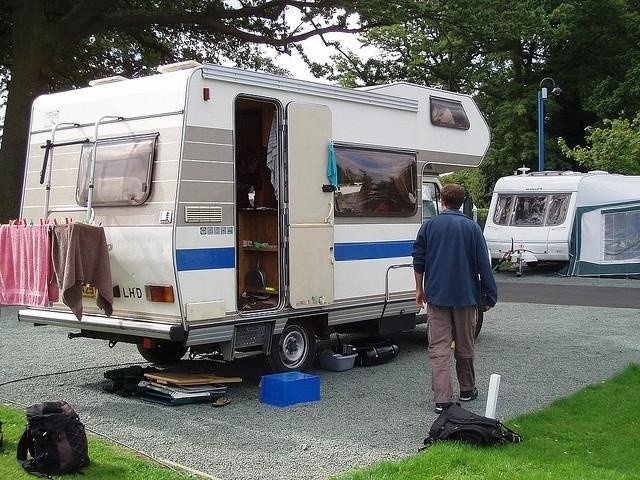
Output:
[537,77,563,172]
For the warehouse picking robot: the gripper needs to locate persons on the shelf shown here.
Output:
[411,182,498,415]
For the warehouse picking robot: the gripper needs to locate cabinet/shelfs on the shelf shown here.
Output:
[238,207,278,302]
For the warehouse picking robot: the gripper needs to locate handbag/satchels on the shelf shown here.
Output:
[16,398,92,480]
[424,404,521,448]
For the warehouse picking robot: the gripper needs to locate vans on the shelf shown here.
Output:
[16,60,491,374]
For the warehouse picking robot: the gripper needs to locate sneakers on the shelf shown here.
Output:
[434,400,460,413]
[459,386,478,401]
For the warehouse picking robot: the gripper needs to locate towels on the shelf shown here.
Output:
[0,223,59,308]
[52,222,114,322]
